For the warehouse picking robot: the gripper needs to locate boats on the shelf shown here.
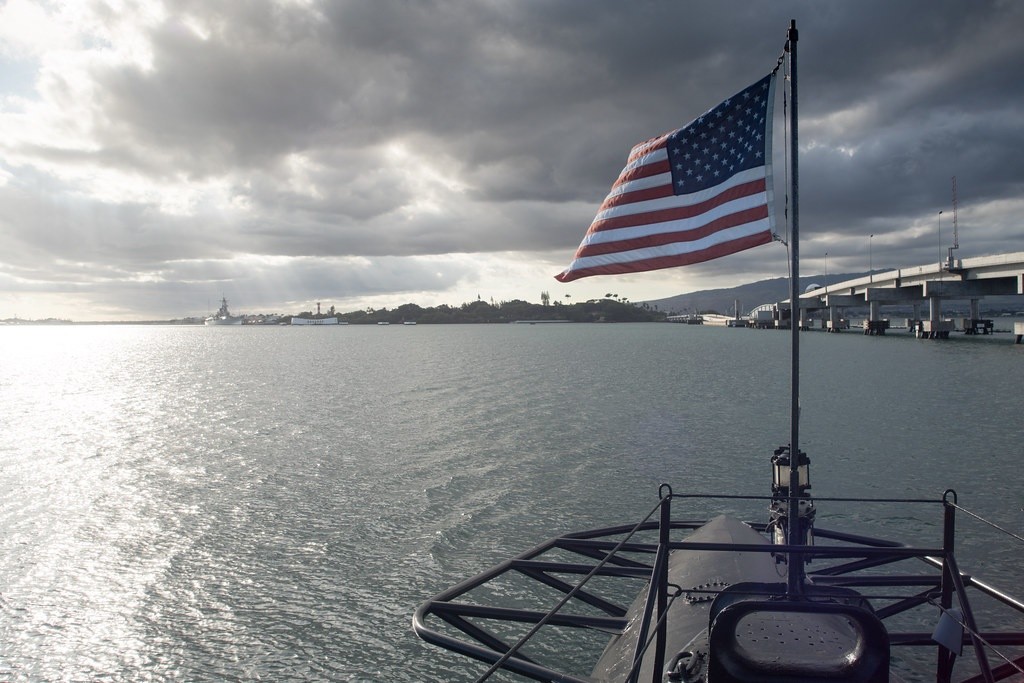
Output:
[204,296,244,325]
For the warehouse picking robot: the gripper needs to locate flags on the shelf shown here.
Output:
[554,68,778,282]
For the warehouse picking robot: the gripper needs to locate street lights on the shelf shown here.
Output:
[825,252,827,289]
[870,235,873,283]
[939,210,942,271]
